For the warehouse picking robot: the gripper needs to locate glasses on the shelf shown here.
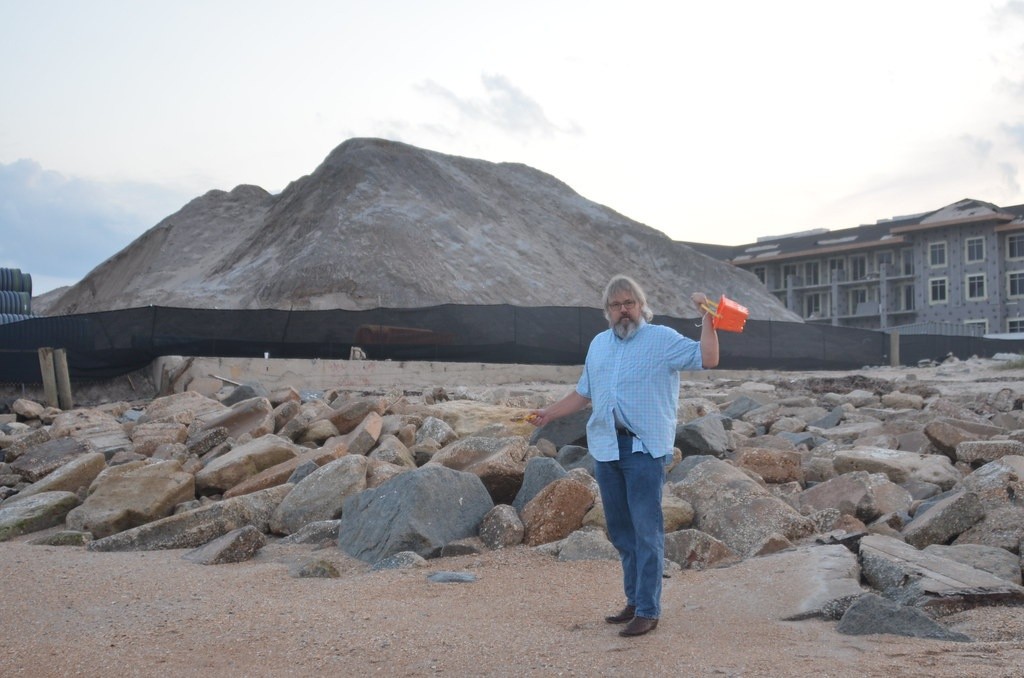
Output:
[607,299,636,311]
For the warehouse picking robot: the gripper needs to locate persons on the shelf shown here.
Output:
[526,274,719,636]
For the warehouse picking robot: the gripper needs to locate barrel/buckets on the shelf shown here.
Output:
[701,295,748,333]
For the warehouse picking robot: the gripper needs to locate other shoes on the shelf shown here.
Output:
[619,617,659,637]
[605,605,635,623]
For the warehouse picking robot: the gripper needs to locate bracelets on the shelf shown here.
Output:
[695,310,711,327]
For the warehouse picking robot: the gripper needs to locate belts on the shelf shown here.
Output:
[615,428,636,436]
